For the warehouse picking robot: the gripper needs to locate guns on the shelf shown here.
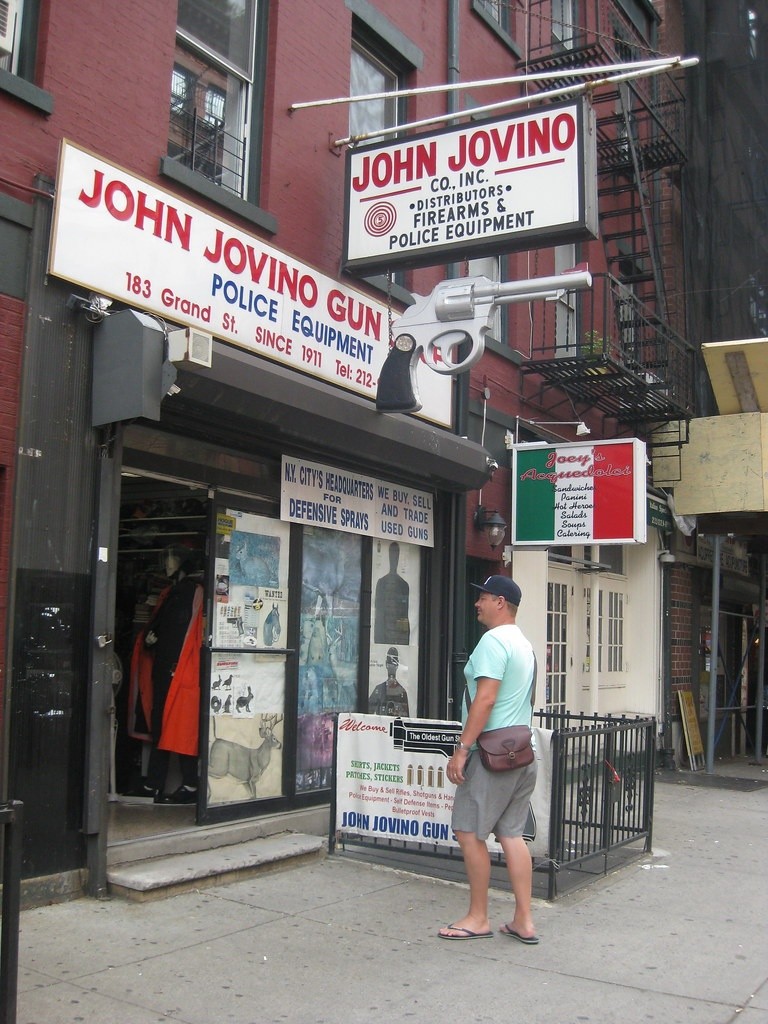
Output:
[375,261,593,414]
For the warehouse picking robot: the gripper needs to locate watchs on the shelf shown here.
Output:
[455,741,471,752]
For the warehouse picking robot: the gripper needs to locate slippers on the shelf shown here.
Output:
[498,924,539,944]
[438,923,493,939]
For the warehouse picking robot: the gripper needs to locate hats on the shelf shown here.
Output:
[471,575,521,606]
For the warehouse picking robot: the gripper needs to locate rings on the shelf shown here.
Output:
[452,774,457,776]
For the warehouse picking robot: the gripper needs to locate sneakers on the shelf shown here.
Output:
[155,786,197,805]
[117,786,155,804]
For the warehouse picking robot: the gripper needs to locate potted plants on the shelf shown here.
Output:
[580,330,611,375]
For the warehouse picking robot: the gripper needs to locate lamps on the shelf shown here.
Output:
[576,424,590,437]
[474,506,508,551]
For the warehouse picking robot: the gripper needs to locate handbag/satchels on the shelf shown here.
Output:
[476,725,534,772]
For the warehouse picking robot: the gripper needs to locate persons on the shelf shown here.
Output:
[437,575,540,944]
[128,540,201,805]
